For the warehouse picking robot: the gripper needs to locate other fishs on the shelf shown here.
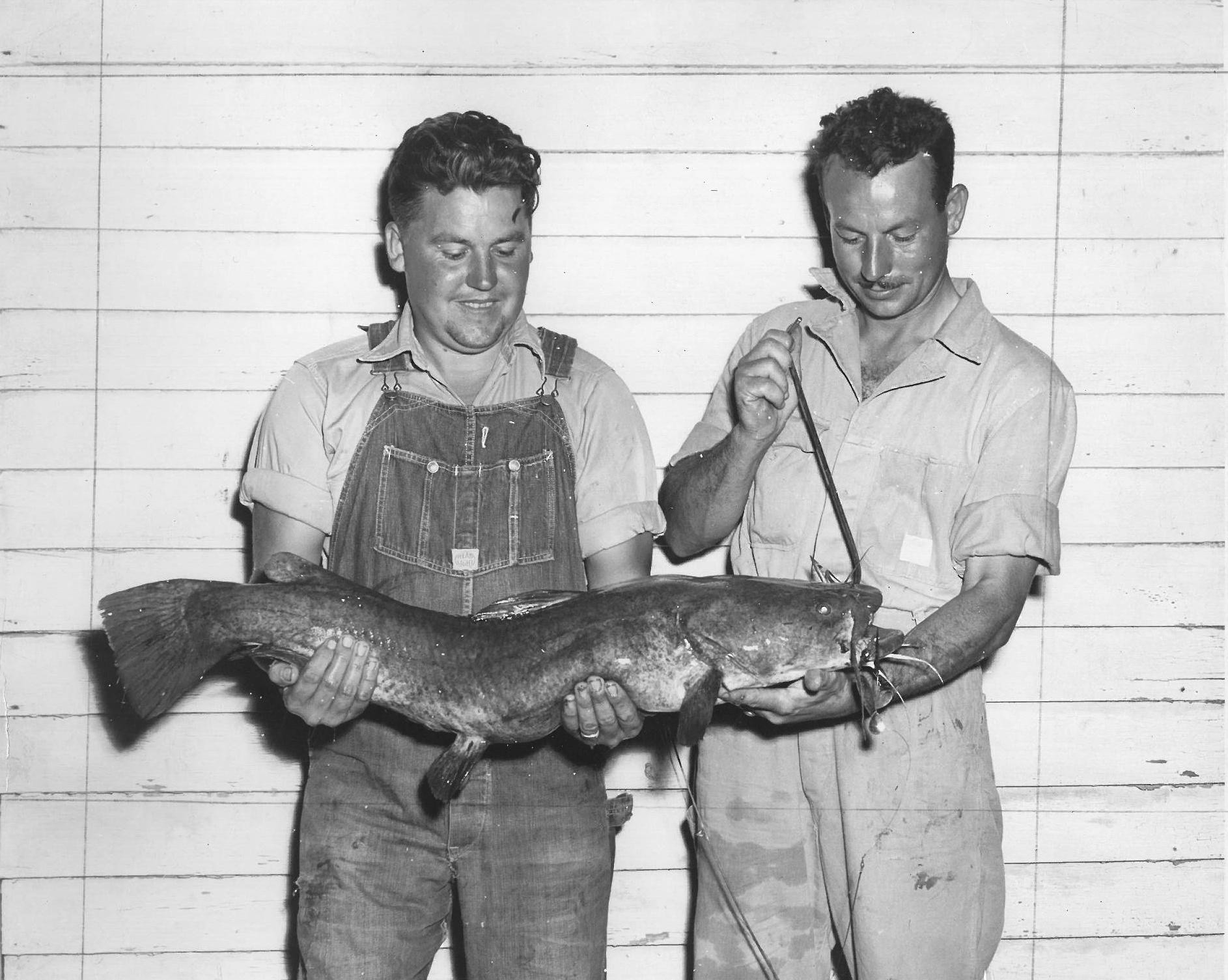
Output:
[99,570,904,808]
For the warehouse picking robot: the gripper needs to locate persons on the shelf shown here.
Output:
[657,88,1077,980]
[239,109,667,980]
